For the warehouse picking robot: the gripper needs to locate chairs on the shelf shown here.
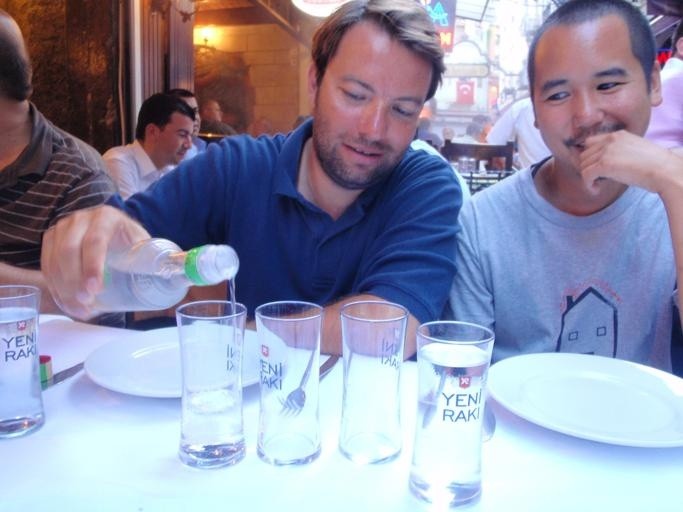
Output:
[442,139,513,193]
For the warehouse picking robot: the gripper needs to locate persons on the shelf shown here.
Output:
[169,88,207,154]
[199,98,237,136]
[641,19,683,158]
[0,7,125,329]
[484,97,551,169]
[441,0,683,375]
[38,0,463,362]
[100,93,195,203]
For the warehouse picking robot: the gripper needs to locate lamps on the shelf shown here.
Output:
[174,1,196,22]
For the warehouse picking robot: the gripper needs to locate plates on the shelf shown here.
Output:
[84,323,288,398]
[487,352,683,448]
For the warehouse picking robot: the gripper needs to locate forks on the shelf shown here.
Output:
[279,339,317,418]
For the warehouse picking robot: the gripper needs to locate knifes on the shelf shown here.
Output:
[53,358,84,386]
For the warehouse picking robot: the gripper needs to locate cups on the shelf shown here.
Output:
[175,301,248,470]
[409,321,495,507]
[338,301,409,466]
[255,299,324,467]
[0,285,46,441]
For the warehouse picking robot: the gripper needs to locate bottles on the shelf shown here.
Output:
[93,236,241,312]
